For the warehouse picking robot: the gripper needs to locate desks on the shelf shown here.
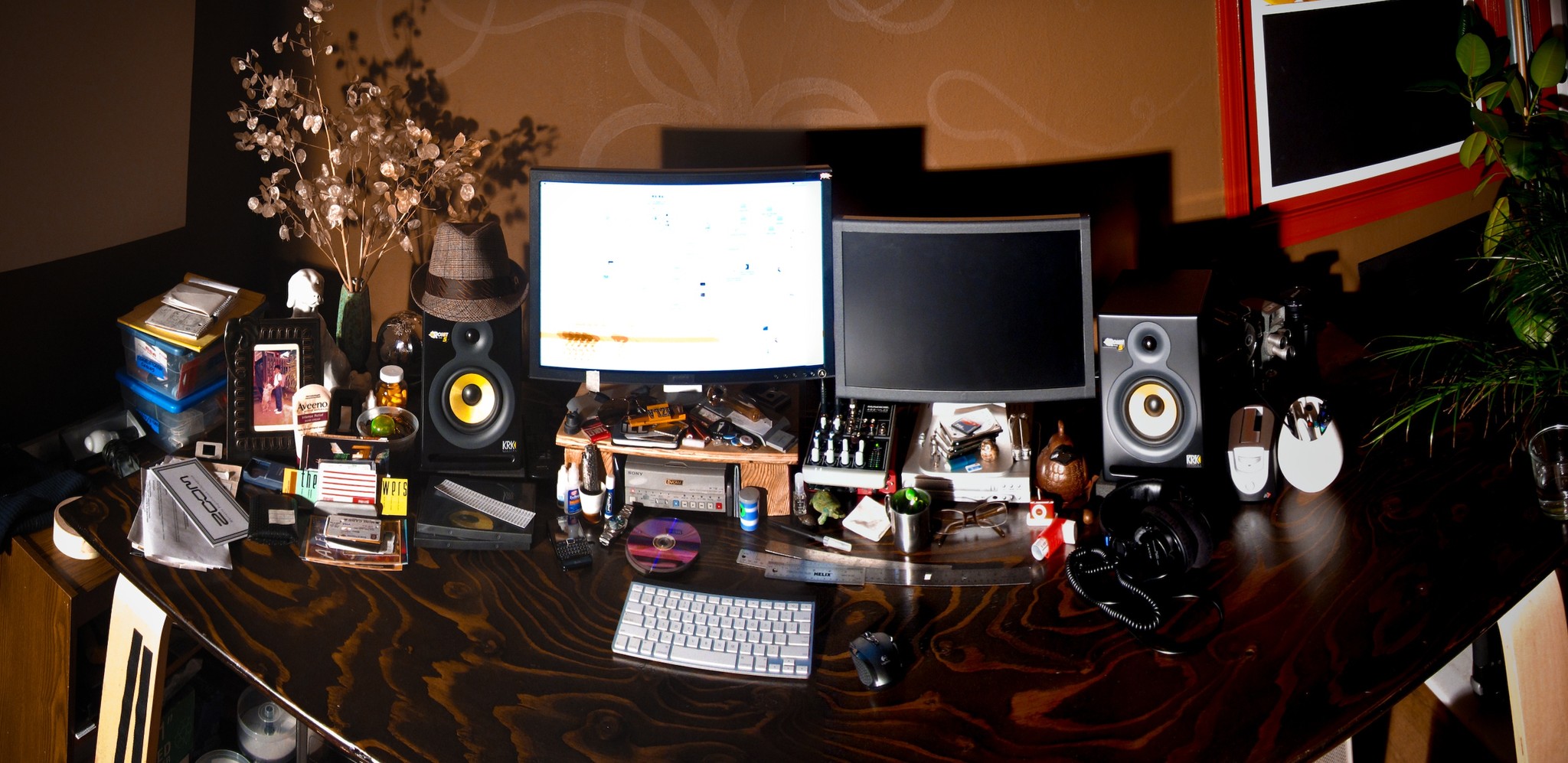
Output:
[58,268,1563,763]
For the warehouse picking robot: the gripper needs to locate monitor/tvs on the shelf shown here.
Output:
[530,164,834,446]
[833,218,1096,479]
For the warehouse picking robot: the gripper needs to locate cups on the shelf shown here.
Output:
[884,487,931,554]
[578,480,606,524]
[1278,396,1344,493]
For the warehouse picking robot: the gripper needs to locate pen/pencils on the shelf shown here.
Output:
[770,519,852,551]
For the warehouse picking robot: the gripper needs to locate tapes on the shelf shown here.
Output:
[53,495,101,560]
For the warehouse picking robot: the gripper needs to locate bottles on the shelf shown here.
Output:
[556,463,582,515]
[375,365,408,411]
[362,390,377,413]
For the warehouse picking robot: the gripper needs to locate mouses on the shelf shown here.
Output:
[849,631,908,691]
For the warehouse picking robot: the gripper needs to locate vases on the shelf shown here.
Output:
[335,278,376,364]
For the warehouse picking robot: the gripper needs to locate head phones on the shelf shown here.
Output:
[1095,477,1222,585]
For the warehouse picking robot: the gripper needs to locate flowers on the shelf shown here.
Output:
[223,0,505,289]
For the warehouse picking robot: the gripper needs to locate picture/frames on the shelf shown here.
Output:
[226,317,324,455]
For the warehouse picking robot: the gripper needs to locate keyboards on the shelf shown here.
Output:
[611,581,816,678]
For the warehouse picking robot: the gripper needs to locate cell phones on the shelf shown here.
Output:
[546,514,595,571]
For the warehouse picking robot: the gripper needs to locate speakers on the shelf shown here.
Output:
[1098,313,1209,483]
[421,310,526,479]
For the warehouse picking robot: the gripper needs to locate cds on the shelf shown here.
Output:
[236,701,297,763]
[626,517,702,575]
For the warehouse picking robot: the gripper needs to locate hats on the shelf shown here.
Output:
[410,218,528,323]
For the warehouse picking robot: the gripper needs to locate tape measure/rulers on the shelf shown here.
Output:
[765,539,952,569]
[735,549,1033,587]
[764,562,866,586]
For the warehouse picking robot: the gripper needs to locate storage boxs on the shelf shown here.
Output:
[116,274,240,400]
[111,368,228,456]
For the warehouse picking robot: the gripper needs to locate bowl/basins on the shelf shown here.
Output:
[356,406,419,456]
